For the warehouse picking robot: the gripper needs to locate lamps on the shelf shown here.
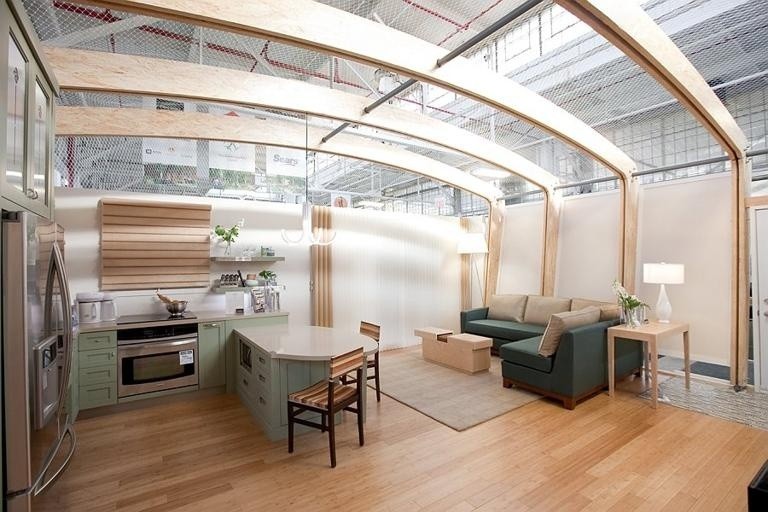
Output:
[458,233,489,306]
[644,262,684,324]
[279,111,337,249]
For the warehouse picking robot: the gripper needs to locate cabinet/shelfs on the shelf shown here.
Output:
[0,0,62,222]
[198,316,288,393]
[78,330,117,409]
[234,325,378,442]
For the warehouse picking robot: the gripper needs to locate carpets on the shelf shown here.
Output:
[639,354,768,435]
[347,350,546,431]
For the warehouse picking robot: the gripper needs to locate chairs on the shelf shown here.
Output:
[286,320,380,467]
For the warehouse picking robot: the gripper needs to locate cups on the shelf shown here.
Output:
[76,292,116,323]
[246,274,256,280]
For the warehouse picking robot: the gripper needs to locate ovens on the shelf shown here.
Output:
[117,339,199,404]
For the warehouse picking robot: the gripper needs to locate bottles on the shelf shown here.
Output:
[219,274,239,287]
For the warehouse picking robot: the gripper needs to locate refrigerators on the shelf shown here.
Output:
[3,207,77,512]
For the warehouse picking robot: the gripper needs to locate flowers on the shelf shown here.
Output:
[612,279,641,307]
[211,219,245,244]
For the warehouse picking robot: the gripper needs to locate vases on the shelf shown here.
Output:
[223,243,232,257]
[623,307,641,330]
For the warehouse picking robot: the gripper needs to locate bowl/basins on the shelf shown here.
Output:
[245,280,258,286]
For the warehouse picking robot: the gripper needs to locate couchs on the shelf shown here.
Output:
[460,293,644,410]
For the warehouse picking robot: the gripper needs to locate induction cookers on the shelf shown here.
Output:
[116,310,197,341]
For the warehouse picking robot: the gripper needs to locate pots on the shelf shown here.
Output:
[165,301,187,317]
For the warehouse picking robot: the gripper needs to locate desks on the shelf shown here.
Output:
[607,320,692,408]
